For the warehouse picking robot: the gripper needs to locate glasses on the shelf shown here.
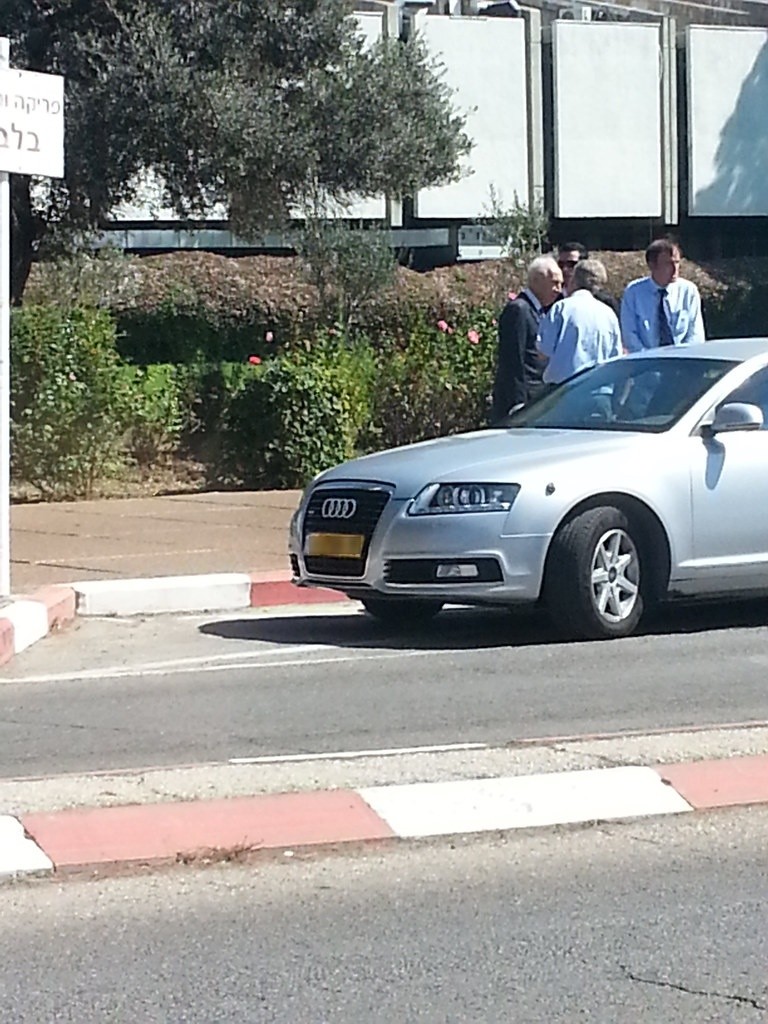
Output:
[557,259,579,268]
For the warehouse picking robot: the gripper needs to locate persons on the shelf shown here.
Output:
[546,242,628,346]
[619,240,705,351]
[492,256,564,424]
[535,260,622,418]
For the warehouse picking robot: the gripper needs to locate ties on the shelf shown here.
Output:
[656,288,676,345]
[537,306,546,320]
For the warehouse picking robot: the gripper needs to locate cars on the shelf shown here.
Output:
[287,337,767,641]
